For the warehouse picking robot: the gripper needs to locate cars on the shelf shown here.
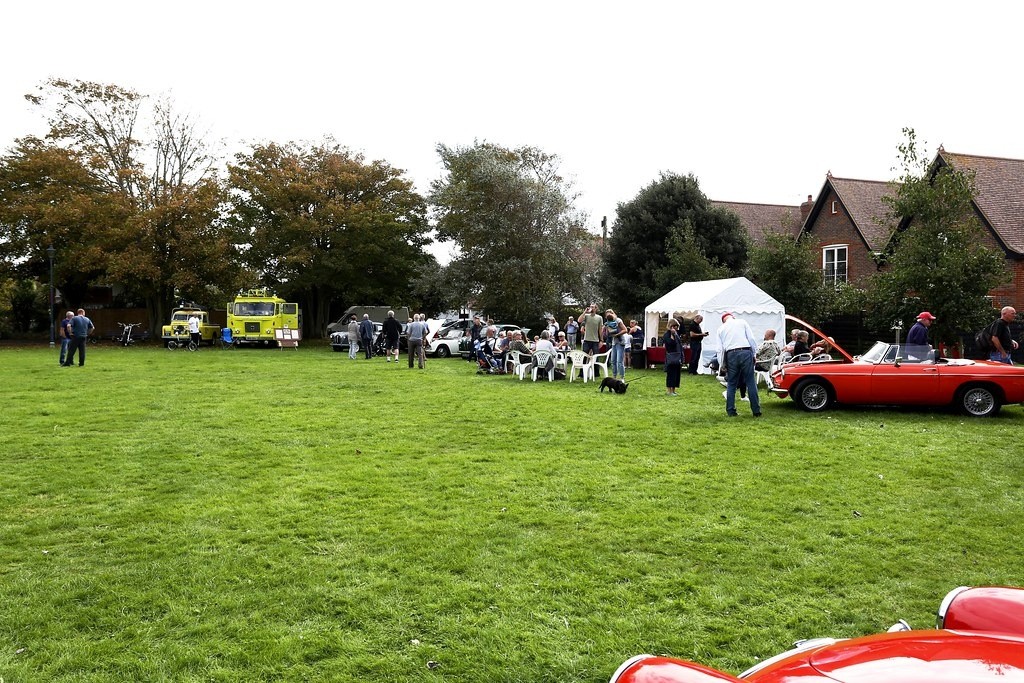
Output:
[375,321,407,355]
[768,314,1024,417]
[330,320,383,352]
[425,316,529,358]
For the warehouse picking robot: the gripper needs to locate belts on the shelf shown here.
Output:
[726,347,749,353]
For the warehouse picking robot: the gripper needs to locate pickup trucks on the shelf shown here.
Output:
[161,307,220,346]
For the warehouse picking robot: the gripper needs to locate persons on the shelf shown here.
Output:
[62,309,96,366]
[360,314,374,360]
[663,315,709,396]
[404,313,430,369]
[989,306,1019,365]
[463,302,643,383]
[382,310,402,363]
[59,311,76,365]
[717,329,835,401]
[716,314,763,416]
[188,311,200,351]
[904,312,937,361]
[347,315,359,360]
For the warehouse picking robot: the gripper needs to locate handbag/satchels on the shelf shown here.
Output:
[666,352,681,366]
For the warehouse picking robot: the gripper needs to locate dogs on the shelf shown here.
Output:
[599,377,629,394]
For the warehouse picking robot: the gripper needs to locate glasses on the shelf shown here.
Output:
[558,336,562,338]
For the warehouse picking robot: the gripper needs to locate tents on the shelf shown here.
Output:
[644,276,786,375]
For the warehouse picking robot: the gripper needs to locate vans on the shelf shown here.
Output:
[326,304,410,335]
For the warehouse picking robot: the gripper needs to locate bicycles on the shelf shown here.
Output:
[168,330,198,351]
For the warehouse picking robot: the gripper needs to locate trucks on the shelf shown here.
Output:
[226,288,302,345]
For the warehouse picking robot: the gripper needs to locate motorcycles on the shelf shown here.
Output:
[117,322,142,347]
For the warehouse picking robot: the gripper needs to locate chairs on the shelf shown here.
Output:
[753,349,793,389]
[221,328,238,350]
[503,345,612,383]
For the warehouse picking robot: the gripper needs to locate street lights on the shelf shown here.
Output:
[46,243,58,348]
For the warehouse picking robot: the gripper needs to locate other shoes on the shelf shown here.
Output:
[715,376,726,382]
[674,392,680,396]
[720,382,728,388]
[387,361,390,363]
[395,359,398,362]
[722,390,727,400]
[668,393,677,396]
[741,396,750,401]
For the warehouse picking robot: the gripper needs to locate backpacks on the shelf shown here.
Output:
[975,320,1006,352]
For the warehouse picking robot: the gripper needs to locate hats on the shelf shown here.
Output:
[919,312,936,320]
[722,313,734,322]
